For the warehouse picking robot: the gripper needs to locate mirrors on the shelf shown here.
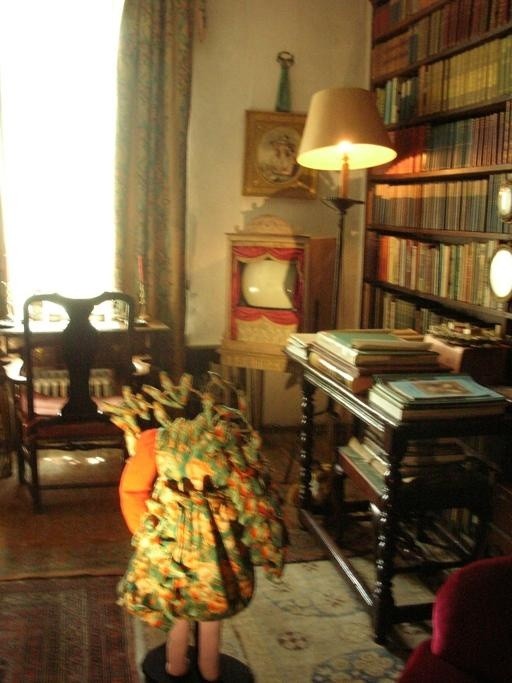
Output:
[484,240,512,301]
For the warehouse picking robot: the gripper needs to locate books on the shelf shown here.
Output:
[373,0,512,330]
[285,327,505,495]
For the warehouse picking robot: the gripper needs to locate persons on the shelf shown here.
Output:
[116,401,289,682]
[256,128,303,184]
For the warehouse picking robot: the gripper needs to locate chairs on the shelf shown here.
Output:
[12,294,140,501]
[394,552,511,682]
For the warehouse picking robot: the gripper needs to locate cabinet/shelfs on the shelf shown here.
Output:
[339,1,512,338]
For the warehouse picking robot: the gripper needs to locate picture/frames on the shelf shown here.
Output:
[239,107,319,199]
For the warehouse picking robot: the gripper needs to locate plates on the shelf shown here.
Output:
[0,311,151,328]
[424,324,502,347]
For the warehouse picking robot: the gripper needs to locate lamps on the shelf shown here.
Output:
[294,88,397,511]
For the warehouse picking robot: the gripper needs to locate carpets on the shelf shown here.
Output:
[119,550,438,682]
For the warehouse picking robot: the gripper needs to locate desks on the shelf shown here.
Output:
[0,317,173,473]
[294,330,502,641]
